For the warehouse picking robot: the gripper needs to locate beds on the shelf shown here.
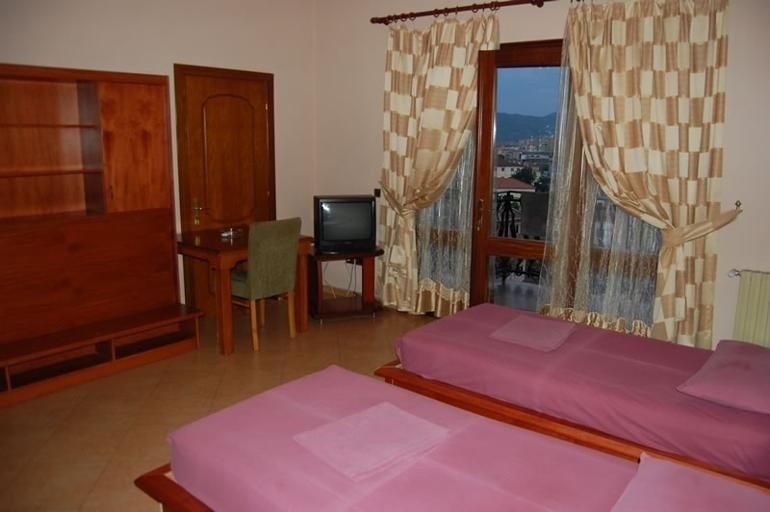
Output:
[137,362,769,512]
[368,301,770,487]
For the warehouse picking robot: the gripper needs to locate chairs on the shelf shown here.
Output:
[230,217,301,351]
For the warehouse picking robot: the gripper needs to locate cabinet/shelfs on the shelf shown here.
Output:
[0,62,205,403]
[310,246,384,319]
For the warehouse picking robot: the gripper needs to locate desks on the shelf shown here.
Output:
[176,225,314,354]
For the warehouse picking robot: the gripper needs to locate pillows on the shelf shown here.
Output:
[677,336,770,415]
[604,449,769,511]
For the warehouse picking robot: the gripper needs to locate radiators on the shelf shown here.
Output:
[733,270,770,349]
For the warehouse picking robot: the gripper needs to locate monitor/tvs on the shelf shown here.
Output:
[314,194,376,254]
